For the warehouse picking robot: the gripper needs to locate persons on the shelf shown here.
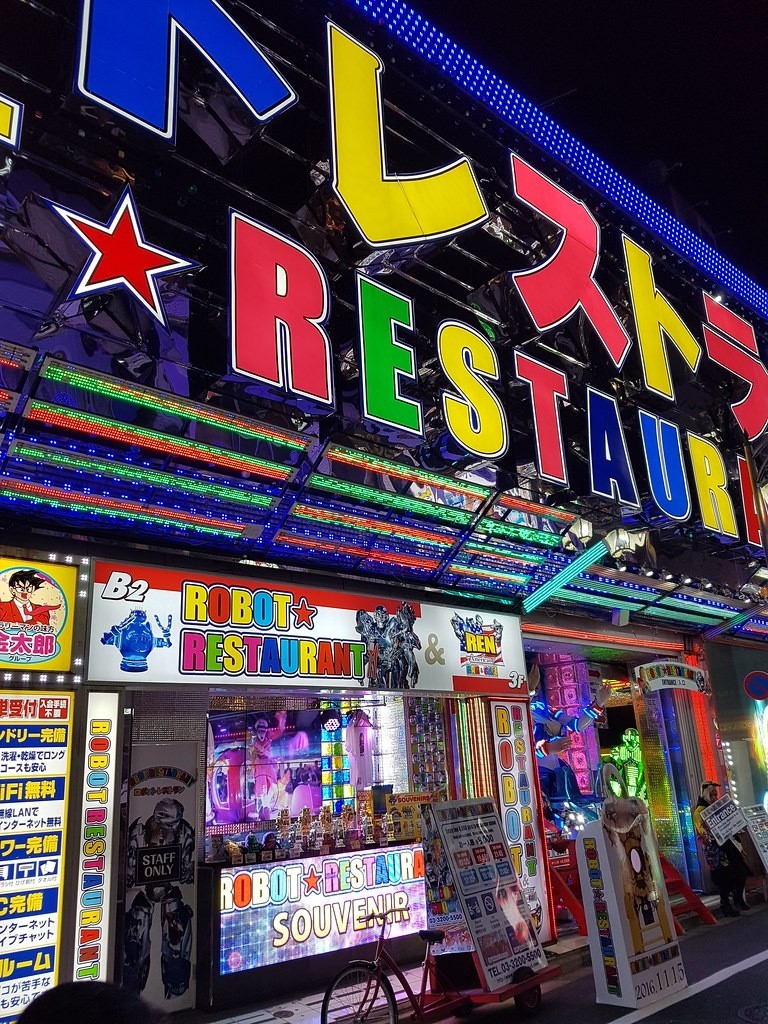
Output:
[693,780,750,917]
[15,981,177,1024]
[247,710,321,795]
[526,664,611,804]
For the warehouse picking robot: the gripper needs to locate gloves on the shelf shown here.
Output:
[733,832,742,843]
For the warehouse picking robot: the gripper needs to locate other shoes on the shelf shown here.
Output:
[720,903,740,917]
[733,895,751,910]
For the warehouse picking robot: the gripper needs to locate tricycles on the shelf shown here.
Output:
[321,907,561,1024]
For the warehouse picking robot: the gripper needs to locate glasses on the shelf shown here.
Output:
[709,793,718,797]
